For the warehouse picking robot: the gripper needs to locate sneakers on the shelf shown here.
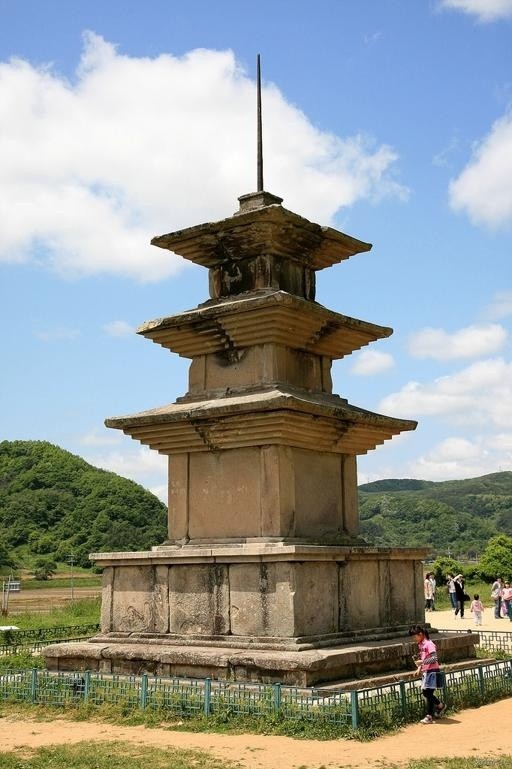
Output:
[420,714,433,724]
[425,606,464,619]
[434,703,447,717]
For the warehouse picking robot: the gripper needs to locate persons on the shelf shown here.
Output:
[408,624,447,724]
[424,571,512,625]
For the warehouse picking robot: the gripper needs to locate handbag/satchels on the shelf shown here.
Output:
[491,589,500,601]
[463,592,470,601]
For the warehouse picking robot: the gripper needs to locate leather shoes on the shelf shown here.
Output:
[495,614,503,618]
